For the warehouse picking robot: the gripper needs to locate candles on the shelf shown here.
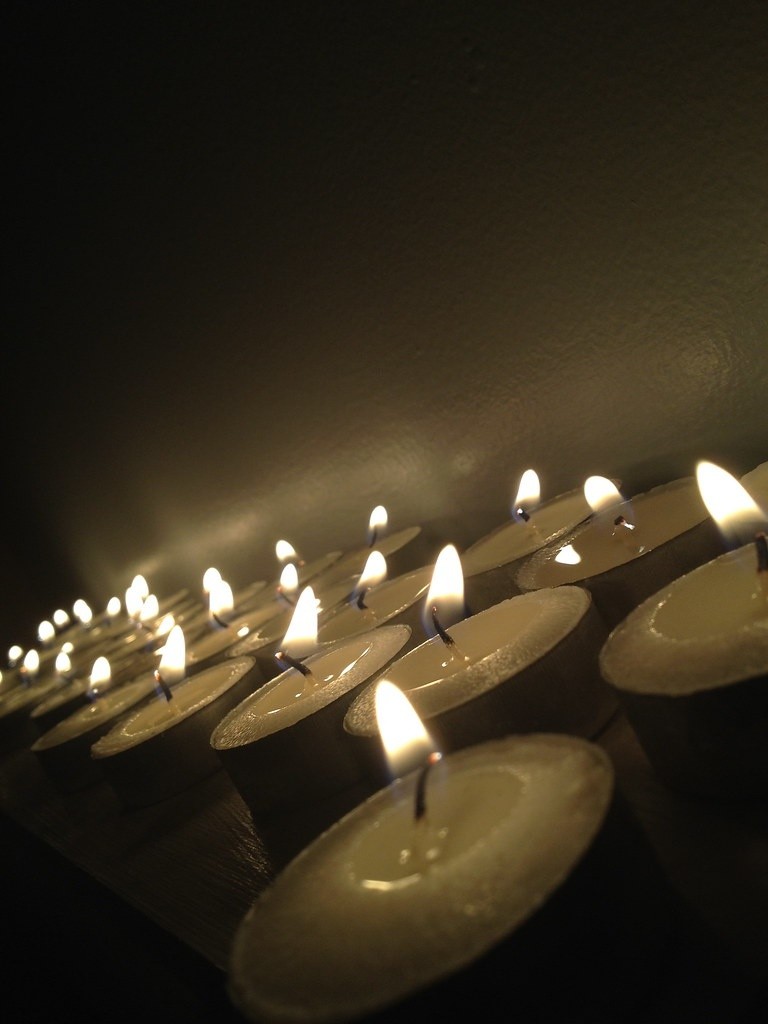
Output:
[343,544,617,773]
[91,625,263,810]
[32,657,166,786]
[212,587,414,817]
[329,501,428,574]
[460,469,625,613]
[517,475,728,633]
[317,551,456,658]
[231,566,376,666]
[257,537,348,606]
[225,678,737,1024]
[599,460,768,806]
[2,542,266,726]
